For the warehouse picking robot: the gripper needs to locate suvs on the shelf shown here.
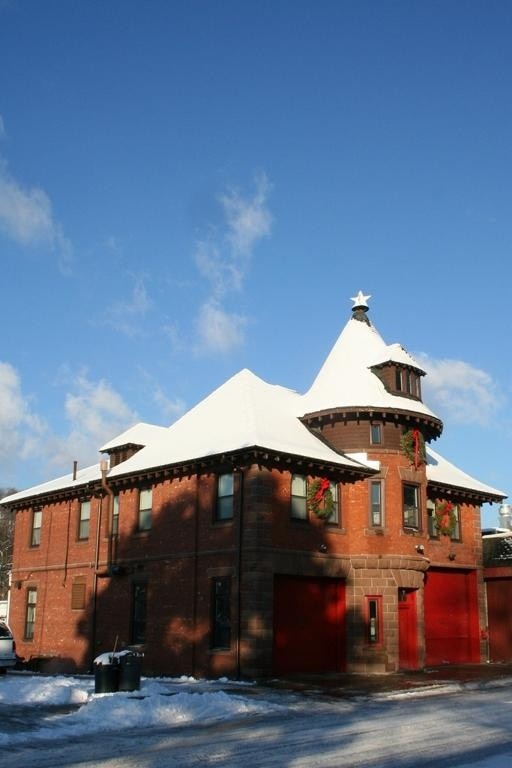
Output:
[0,618,17,676]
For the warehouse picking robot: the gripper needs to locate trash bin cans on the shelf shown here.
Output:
[93,652,120,693]
[119,650,142,691]
